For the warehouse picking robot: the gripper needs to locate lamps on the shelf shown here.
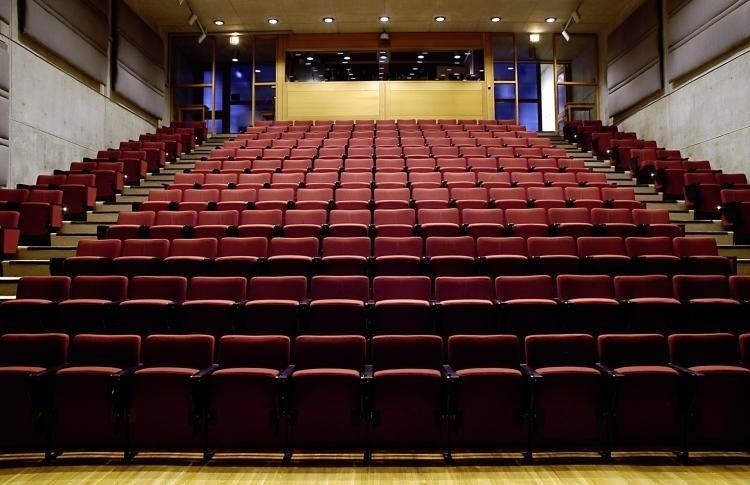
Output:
[197,34,206,44]
[188,12,198,26]
[571,10,581,24]
[561,29,571,42]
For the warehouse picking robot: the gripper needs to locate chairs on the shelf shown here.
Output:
[673,237,718,257]
[527,186,563,199]
[476,255,533,275]
[653,159,697,202]
[95,224,146,240]
[591,132,616,161]
[117,210,155,225]
[143,224,188,240]
[152,210,198,227]
[233,299,302,335]
[714,174,750,189]
[284,333,373,469]
[682,255,739,275]
[321,237,373,255]
[453,199,491,209]
[489,187,526,199]
[225,224,280,237]
[595,333,703,461]
[526,236,576,257]
[267,236,319,257]
[442,334,542,465]
[107,256,162,277]
[158,255,211,276]
[467,157,504,171]
[492,275,554,301]
[339,158,376,171]
[245,275,309,303]
[505,208,547,224]
[184,189,218,201]
[619,298,685,336]
[198,335,295,469]
[52,174,96,217]
[166,142,195,161]
[298,298,370,334]
[513,147,552,159]
[643,224,687,237]
[109,150,147,187]
[459,146,492,157]
[69,275,128,301]
[246,199,288,209]
[590,207,631,224]
[487,147,518,158]
[573,236,625,257]
[570,198,608,208]
[170,237,219,257]
[373,236,424,257]
[681,173,733,220]
[425,235,476,256]
[682,161,723,209]
[307,158,344,171]
[147,189,182,202]
[506,223,554,237]
[632,139,658,149]
[669,334,749,460]
[124,275,189,302]
[200,148,234,161]
[462,208,505,224]
[631,209,670,225]
[369,254,426,275]
[284,148,319,159]
[530,255,583,276]
[728,275,749,300]
[417,207,461,223]
[54,299,113,337]
[556,158,589,171]
[306,275,371,302]
[368,199,412,210]
[0,187,29,226]
[412,200,451,209]
[374,187,410,200]
[0,210,20,261]
[335,171,373,188]
[435,274,495,303]
[288,200,331,209]
[452,187,488,200]
[213,159,252,174]
[372,158,409,170]
[498,159,533,171]
[373,208,417,225]
[369,276,433,302]
[597,126,618,132]
[613,275,671,298]
[275,159,313,172]
[557,274,615,301]
[364,299,434,336]
[239,208,283,224]
[207,199,250,210]
[195,210,239,226]
[112,300,175,335]
[312,255,371,276]
[16,174,67,192]
[161,172,204,189]
[322,223,371,237]
[720,189,750,246]
[8,189,63,244]
[608,199,646,209]
[737,333,750,362]
[598,222,642,238]
[120,237,169,256]
[313,147,348,159]
[582,120,602,127]
[475,236,526,255]
[558,296,624,335]
[478,172,517,187]
[296,188,333,201]
[654,149,689,161]
[205,256,262,277]
[182,224,235,238]
[75,238,122,257]
[82,150,121,162]
[187,275,247,303]
[131,200,172,211]
[220,188,258,201]
[511,171,548,187]
[409,171,445,187]
[610,132,637,166]
[435,157,472,171]
[373,146,405,157]
[423,255,479,275]
[412,187,450,200]
[531,199,569,208]
[183,160,221,174]
[498,299,563,336]
[412,222,462,237]
[431,298,502,337]
[672,275,729,300]
[611,138,635,173]
[258,188,294,201]
[55,334,141,461]
[159,120,207,142]
[342,147,376,158]
[243,159,282,173]
[528,159,559,171]
[193,174,237,189]
[364,335,459,463]
[331,200,372,209]
[335,187,372,201]
[430,146,464,158]
[401,146,434,158]
[632,255,684,274]
[0,298,58,334]
[107,141,142,151]
[563,119,582,143]
[48,256,110,276]
[544,172,580,186]
[113,334,217,466]
[257,254,318,276]
[584,255,632,275]
[405,158,441,171]
[283,208,327,225]
[519,334,616,460]
[601,187,635,200]
[179,299,241,338]
[565,187,602,200]
[368,224,419,238]
[80,162,124,205]
[325,208,372,224]
[547,208,591,224]
[256,149,291,159]
[136,142,165,175]
[227,173,271,188]
[129,132,159,142]
[464,223,508,237]
[629,148,658,185]
[53,162,96,174]
[577,125,600,153]
[168,200,211,210]
[621,236,672,257]
[542,147,574,159]
[370,171,406,187]
[228,149,263,160]
[491,198,532,209]
[15,275,71,302]
[551,222,597,237]
[219,236,268,257]
[577,172,617,186]
[0,333,71,460]
[443,171,481,187]
[263,172,304,188]
[676,299,746,335]
[299,172,339,188]
[271,224,324,237]
